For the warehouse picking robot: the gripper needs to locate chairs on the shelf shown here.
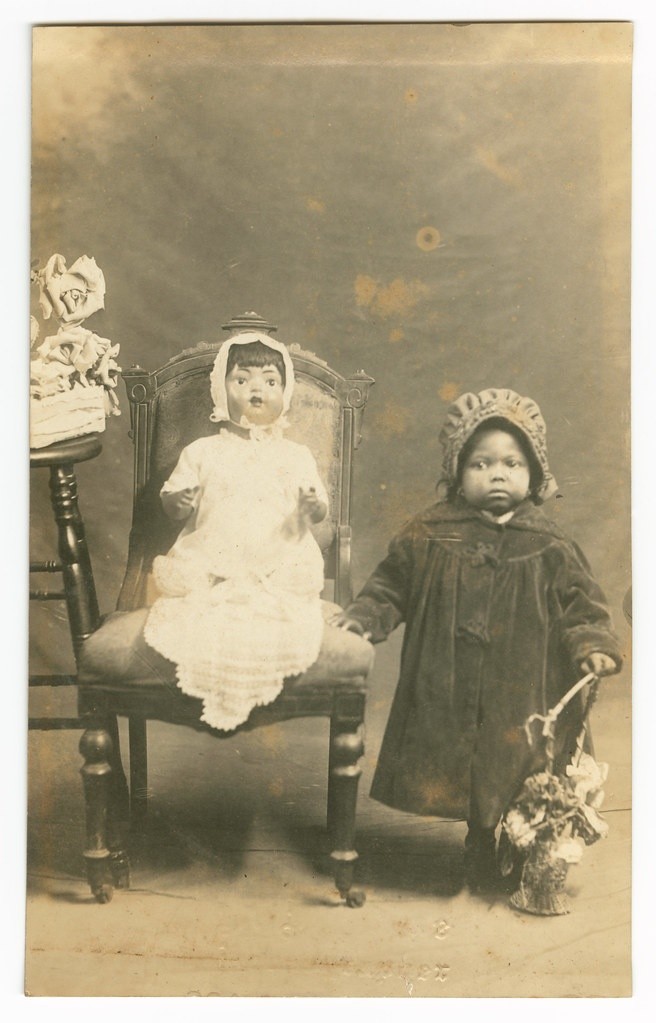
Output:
[75,313,377,906]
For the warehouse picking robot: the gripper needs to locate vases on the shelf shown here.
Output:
[29,385,107,449]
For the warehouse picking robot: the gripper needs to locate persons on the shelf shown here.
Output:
[151,332,329,594]
[324,388,623,894]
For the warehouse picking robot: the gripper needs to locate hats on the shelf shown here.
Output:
[438,387,559,507]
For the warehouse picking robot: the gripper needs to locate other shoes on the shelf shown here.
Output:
[463,828,525,894]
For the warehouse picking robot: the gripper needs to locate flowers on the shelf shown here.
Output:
[30,254,124,399]
[503,750,604,864]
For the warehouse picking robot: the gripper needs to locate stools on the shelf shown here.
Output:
[28,436,130,891]
